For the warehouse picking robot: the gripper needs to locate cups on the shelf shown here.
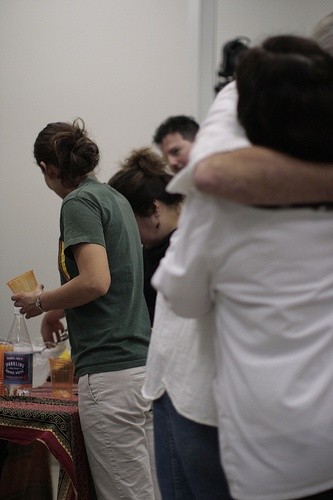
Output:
[48,358,74,401]
[6,269,40,295]
[0,337,14,394]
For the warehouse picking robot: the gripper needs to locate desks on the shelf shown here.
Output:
[0,382,96,500]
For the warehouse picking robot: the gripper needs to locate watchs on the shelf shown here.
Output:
[34,292,47,314]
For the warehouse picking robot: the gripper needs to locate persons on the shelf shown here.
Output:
[151,47,333,500]
[11,122,162,500]
[106,146,187,328]
[153,115,201,174]
[140,35,333,500]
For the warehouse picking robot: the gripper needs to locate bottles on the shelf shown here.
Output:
[3,306,33,397]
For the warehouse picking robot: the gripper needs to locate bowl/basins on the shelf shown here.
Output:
[9,342,67,389]
